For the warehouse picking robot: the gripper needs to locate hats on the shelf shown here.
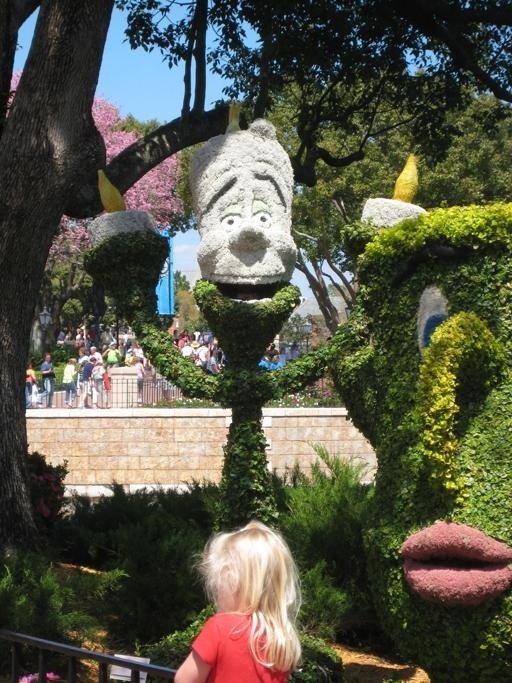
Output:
[191,340,200,347]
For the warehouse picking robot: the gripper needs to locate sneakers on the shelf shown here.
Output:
[36,403,56,408]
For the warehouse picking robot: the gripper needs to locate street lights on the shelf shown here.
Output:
[37,304,50,362]
[303,319,312,353]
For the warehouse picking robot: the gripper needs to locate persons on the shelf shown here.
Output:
[173,520,304,683]
[24,325,304,409]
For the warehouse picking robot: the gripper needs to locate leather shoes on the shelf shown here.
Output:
[65,402,112,409]
